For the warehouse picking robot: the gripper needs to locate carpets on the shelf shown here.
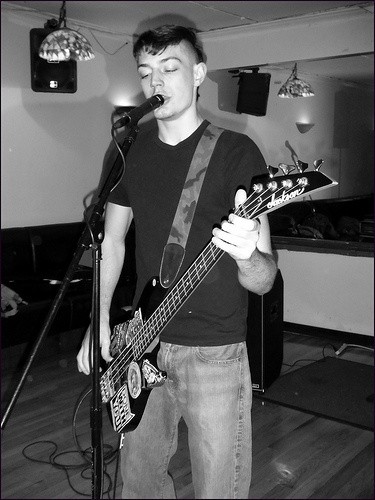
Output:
[256,356,375,430]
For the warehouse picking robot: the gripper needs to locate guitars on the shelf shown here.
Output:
[99,157,341,436]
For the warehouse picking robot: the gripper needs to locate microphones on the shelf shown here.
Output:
[112,94,164,129]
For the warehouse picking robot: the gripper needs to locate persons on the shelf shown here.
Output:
[77,25,278,500]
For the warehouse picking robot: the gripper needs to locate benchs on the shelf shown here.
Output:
[1,224,138,349]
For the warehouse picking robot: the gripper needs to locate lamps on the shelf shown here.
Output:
[295,121,316,134]
[114,105,138,118]
[38,1,96,62]
[278,62,314,98]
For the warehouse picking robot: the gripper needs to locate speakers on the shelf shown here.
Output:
[236,72,271,116]
[29,28,77,93]
[247,269,283,393]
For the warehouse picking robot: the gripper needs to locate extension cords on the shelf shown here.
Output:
[335,342,348,356]
